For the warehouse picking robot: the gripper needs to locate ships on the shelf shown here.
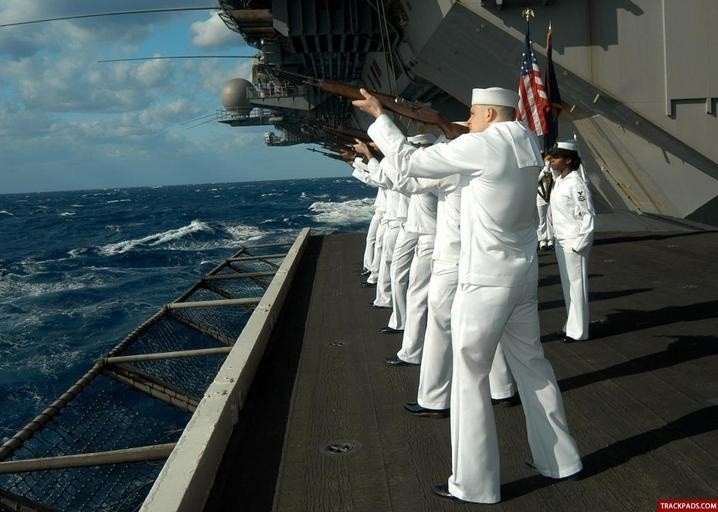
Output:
[0,0,718,512]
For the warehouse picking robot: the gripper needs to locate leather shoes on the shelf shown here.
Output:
[360,269,371,278]
[560,336,578,344]
[367,301,380,308]
[557,330,566,337]
[383,356,419,366]
[359,281,377,289]
[430,484,474,502]
[376,325,404,335]
[491,395,515,408]
[402,402,449,418]
[524,456,537,469]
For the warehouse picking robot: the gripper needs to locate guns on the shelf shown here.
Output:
[261,64,469,140]
[310,136,364,158]
[305,146,347,164]
[273,111,385,169]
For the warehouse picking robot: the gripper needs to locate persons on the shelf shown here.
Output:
[352,87,586,505]
[350,133,439,367]
[550,143,595,341]
[536,155,554,250]
[347,153,410,308]
[365,121,516,416]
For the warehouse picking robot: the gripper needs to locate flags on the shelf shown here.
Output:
[546,31,564,153]
[515,24,551,137]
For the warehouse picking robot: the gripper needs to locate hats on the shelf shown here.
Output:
[407,132,435,144]
[548,141,578,153]
[471,87,519,108]
